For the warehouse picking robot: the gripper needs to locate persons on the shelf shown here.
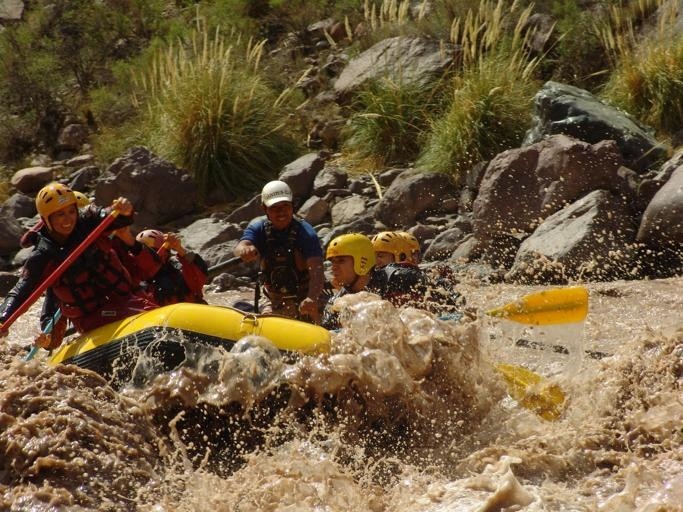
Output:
[235,181,452,331]
[0,184,209,351]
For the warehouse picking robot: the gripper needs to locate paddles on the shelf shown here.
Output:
[432,289,590,326]
[489,362,569,425]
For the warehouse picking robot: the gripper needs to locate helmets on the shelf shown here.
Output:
[35,181,80,233]
[72,189,90,208]
[134,229,168,250]
[372,230,423,267]
[325,233,377,276]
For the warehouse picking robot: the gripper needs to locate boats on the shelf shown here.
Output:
[35,302,486,384]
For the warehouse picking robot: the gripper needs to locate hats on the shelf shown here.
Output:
[260,180,295,208]
